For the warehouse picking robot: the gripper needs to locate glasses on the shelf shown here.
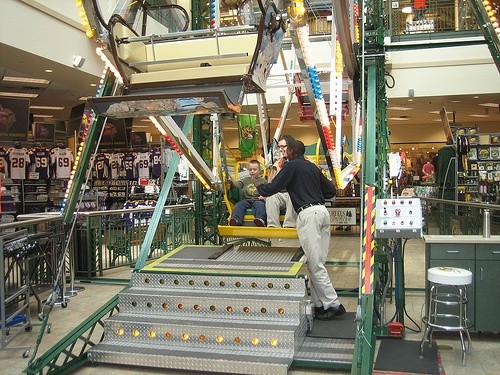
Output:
[278,145,287,149]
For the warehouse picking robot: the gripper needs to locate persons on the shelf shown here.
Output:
[228,160,267,227]
[266,135,314,228]
[254,141,346,320]
[422,162,434,181]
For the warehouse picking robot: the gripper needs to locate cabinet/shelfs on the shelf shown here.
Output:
[424,243,500,333]
[0,138,187,224]
[454,132,500,217]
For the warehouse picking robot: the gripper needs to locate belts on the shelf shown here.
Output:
[297,203,324,215]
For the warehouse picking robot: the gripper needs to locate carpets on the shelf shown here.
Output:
[374,338,440,375]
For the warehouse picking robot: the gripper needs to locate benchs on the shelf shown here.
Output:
[107,221,171,266]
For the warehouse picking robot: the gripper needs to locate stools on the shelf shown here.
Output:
[418,267,473,366]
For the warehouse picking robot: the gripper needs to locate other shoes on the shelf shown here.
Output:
[316,303,347,319]
[254,218,266,227]
[230,218,239,226]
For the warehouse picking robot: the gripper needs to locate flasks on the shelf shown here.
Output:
[483,209,490,239]
